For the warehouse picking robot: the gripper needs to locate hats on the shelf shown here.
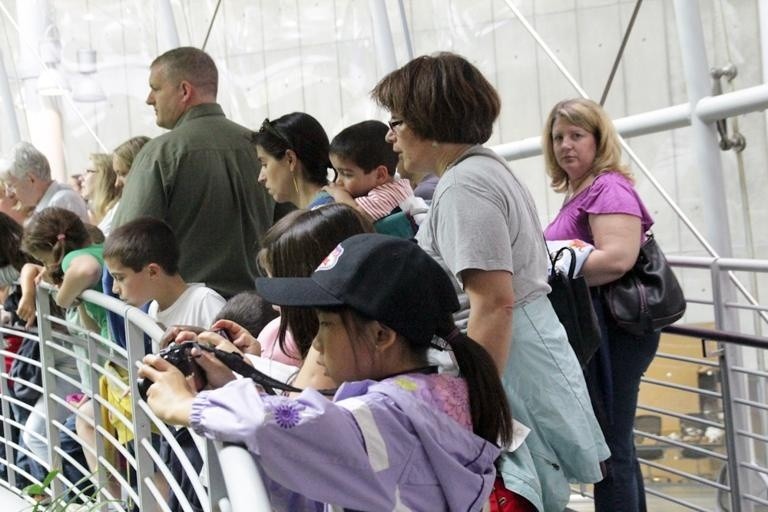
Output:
[255,232,460,334]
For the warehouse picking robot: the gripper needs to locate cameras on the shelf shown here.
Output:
[136,340,205,403]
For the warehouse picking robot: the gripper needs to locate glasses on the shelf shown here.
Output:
[387,119,403,129]
[259,118,288,147]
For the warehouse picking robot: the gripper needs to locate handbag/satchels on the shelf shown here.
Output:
[544,246,602,368]
[600,234,685,335]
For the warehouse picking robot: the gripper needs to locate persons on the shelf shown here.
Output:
[0,47,664,511]
